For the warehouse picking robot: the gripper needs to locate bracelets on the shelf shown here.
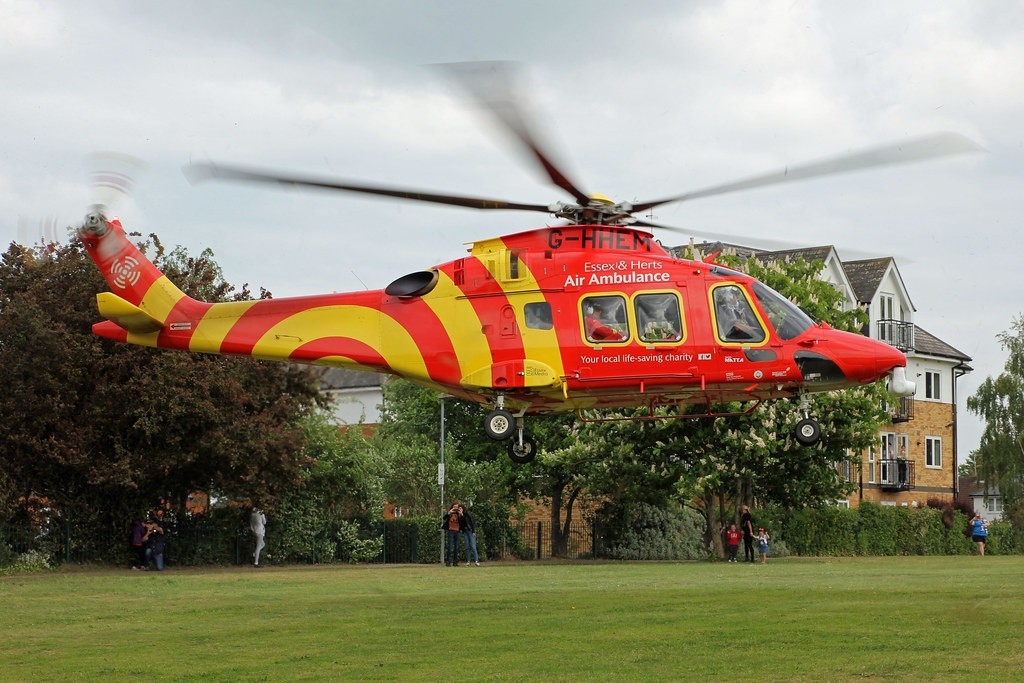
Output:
[750,533,753,534]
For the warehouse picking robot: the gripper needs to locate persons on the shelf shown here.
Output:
[646,301,678,340]
[753,528,770,564]
[740,505,754,564]
[131,520,171,571]
[441,502,464,567]
[249,507,267,568]
[458,504,480,567]
[969,509,990,556]
[583,299,622,341]
[726,524,742,563]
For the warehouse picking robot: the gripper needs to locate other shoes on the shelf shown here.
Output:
[254,564,263,568]
[728,559,731,562]
[453,561,458,566]
[734,558,738,563]
[140,565,151,571]
[751,559,754,563]
[446,560,450,566]
[743,558,750,562]
[131,565,137,570]
[466,561,471,566]
[475,562,480,566]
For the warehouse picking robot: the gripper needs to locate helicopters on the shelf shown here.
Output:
[19,60,989,463]
